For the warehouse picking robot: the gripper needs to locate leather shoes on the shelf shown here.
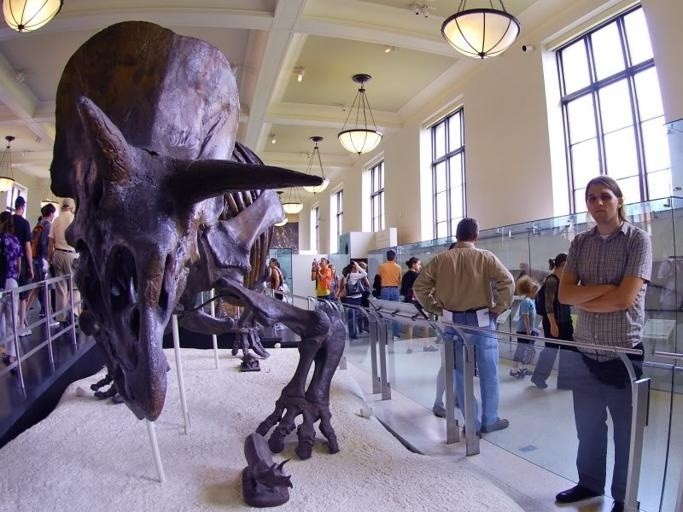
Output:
[556,485,600,503]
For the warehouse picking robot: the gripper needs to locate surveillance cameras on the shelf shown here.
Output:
[521,44,534,52]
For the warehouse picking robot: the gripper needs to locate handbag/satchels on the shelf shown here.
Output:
[534,286,556,316]
[346,280,364,296]
[581,342,643,389]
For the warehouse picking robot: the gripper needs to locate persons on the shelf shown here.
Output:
[516,263,529,281]
[510,275,541,379]
[265,242,461,418]
[0,196,86,376]
[556,175,653,512]
[531,253,575,389]
[411,217,510,439]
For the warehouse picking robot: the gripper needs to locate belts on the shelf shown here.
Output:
[55,248,72,253]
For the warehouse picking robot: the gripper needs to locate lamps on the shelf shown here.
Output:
[272,75,383,226]
[441,0,521,59]
[2,0,64,32]
[0,136,16,191]
[41,168,59,209]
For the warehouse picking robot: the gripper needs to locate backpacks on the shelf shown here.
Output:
[31,221,48,259]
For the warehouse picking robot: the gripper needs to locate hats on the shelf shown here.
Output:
[63,198,73,208]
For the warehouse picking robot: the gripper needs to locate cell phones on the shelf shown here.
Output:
[313,257,316,262]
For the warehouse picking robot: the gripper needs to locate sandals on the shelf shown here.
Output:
[509,368,533,379]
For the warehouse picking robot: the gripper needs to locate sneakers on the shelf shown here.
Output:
[432,403,445,417]
[480,419,508,432]
[530,378,546,388]
